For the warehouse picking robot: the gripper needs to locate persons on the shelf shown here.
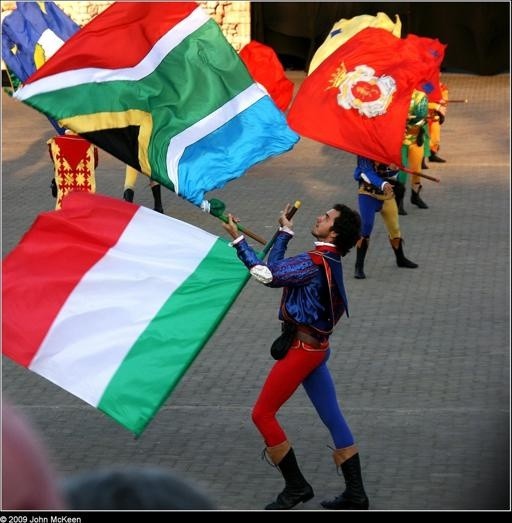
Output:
[220,198,369,508]
[352,69,448,279]
[122,164,165,215]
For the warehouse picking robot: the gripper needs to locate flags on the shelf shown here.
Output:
[285,9,447,169]
[47,133,97,211]
[2,189,267,436]
[12,0,300,220]
[240,38,295,111]
[2,0,82,136]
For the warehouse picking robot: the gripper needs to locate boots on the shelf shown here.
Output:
[421,158,428,169]
[150,186,164,213]
[264,441,314,509]
[394,187,408,215]
[390,237,418,268]
[354,237,370,279]
[320,446,369,509]
[123,188,133,203]
[429,150,446,163]
[411,185,429,209]
